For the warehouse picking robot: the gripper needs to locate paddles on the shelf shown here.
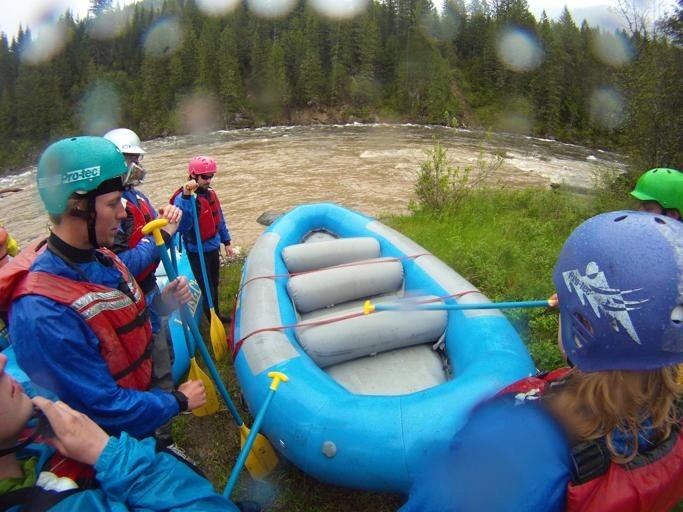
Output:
[142,218,278,483]
[172,237,218,418]
[187,184,228,363]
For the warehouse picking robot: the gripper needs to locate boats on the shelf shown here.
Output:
[232,202,538,495]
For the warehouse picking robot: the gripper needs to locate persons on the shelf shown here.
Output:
[1,354,241,512]
[399,210,681,511]
[104,128,195,464]
[6,135,207,441]
[170,155,234,323]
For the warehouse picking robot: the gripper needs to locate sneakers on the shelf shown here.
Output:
[168,443,195,466]
[220,316,232,323]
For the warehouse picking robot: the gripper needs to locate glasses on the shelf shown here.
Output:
[199,173,215,180]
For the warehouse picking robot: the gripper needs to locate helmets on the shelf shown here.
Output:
[189,156,216,179]
[35,136,130,216]
[104,128,146,157]
[553,210,683,373]
